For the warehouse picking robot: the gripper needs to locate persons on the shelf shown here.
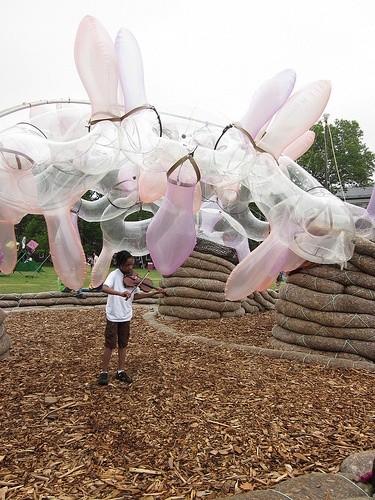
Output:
[97,249,167,386]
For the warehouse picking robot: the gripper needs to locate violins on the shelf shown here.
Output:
[123,270,169,298]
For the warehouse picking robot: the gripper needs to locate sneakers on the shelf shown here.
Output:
[115,370,133,383]
[98,371,108,385]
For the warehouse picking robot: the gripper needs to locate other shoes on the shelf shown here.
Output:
[77,294,86,299]
